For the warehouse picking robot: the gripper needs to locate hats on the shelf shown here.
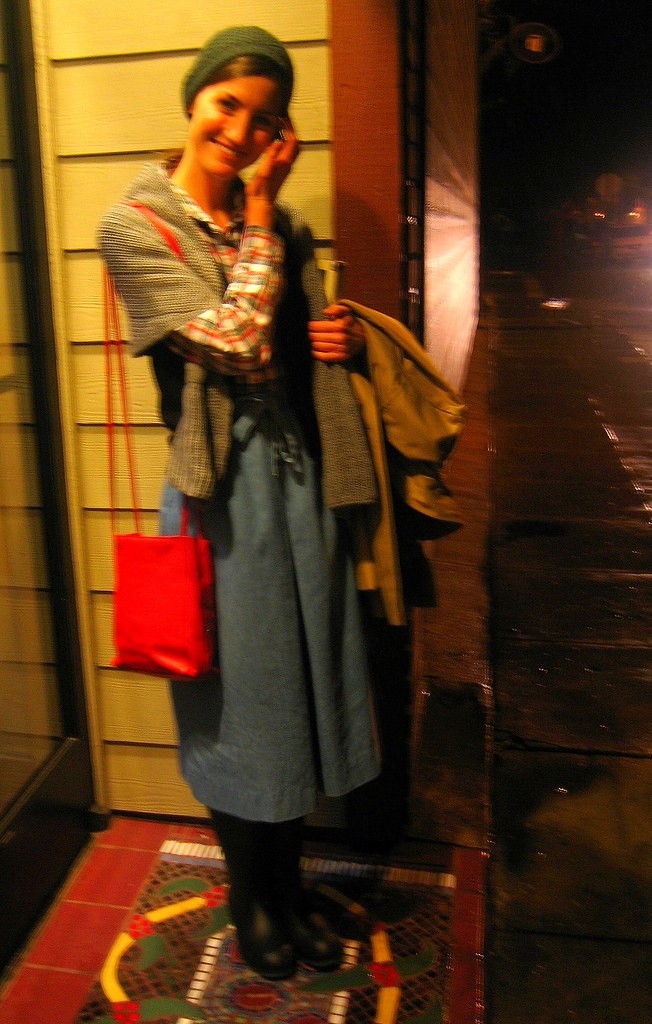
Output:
[180,25,293,122]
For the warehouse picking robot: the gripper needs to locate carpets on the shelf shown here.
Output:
[0,816,490,1024]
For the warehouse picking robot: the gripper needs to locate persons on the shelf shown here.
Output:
[98,26,370,979]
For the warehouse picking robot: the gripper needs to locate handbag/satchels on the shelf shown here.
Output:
[110,532,222,683]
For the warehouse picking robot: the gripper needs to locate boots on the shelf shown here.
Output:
[210,808,294,978]
[258,816,344,968]
